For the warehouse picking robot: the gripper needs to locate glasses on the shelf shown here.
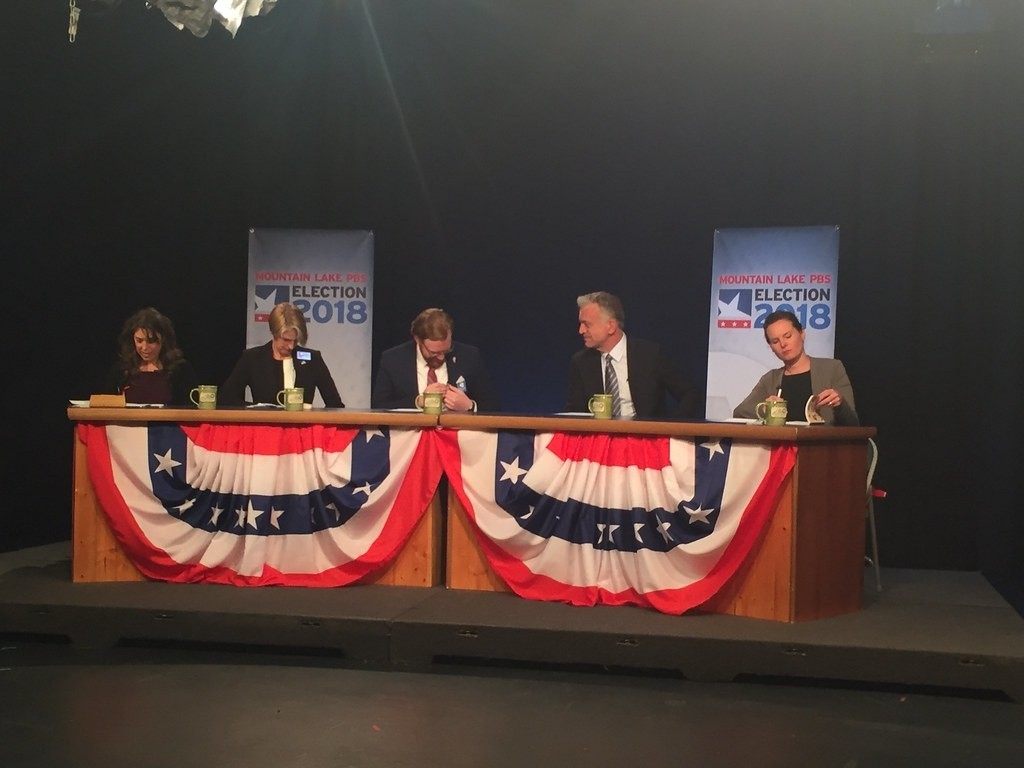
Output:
[420,338,455,357]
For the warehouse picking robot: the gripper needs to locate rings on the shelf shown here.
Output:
[829,402,832,405]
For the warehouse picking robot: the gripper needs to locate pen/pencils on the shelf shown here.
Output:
[776,384,783,398]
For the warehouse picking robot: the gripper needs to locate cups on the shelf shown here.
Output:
[276,388,304,411]
[189,385,217,410]
[755,401,788,425]
[588,394,613,419]
[415,392,443,413]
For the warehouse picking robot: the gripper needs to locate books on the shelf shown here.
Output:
[785,394,825,425]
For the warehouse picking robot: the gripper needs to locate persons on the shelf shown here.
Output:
[733,311,860,426]
[106,308,201,404]
[567,292,671,417]
[217,302,345,408]
[373,308,501,411]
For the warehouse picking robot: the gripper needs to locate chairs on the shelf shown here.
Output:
[865,437,883,591]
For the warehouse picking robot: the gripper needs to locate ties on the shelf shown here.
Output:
[604,354,620,416]
[426,364,437,386]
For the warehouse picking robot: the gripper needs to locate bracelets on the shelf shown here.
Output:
[468,402,475,412]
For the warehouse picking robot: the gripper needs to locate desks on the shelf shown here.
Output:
[68,401,442,589]
[439,409,878,624]
